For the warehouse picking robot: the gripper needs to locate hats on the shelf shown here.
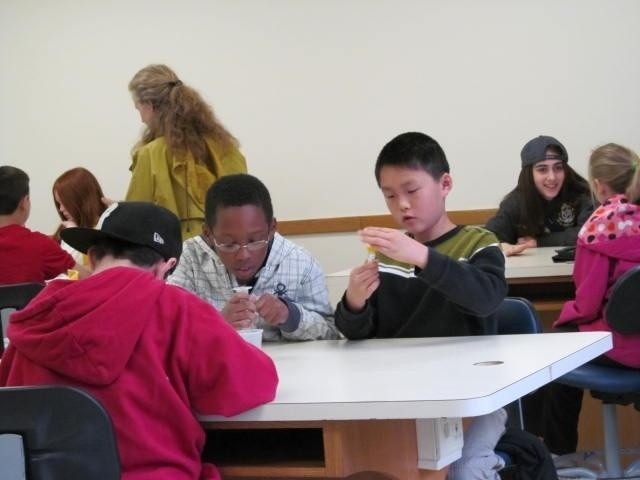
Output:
[60,202,182,275]
[521,136,568,167]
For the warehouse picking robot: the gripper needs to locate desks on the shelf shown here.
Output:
[197,330,614,480]
[325,243,577,333]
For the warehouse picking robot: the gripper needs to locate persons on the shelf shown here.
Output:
[532,141,640,471]
[122,62,250,245]
[331,128,508,479]
[0,164,100,291]
[45,166,113,247]
[167,173,344,346]
[485,134,600,259]
[0,200,283,480]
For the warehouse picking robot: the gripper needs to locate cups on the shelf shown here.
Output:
[236,328,264,350]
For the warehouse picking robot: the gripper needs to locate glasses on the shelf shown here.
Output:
[212,236,269,252]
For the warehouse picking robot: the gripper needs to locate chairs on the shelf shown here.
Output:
[552,266,640,480]
[0,282,45,359]
[0,384,122,480]
[498,297,541,333]
